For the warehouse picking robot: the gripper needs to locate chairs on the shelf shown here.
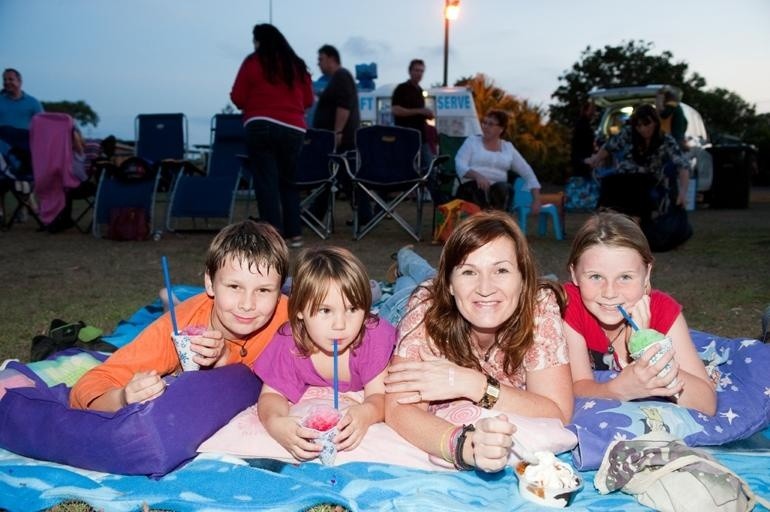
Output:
[164,112,254,234]
[342,123,451,241]
[91,114,190,240]
[0,121,44,231]
[30,112,94,232]
[289,126,339,239]
[431,133,479,234]
[513,178,566,241]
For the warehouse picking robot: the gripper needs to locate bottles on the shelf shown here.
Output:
[152,229,164,242]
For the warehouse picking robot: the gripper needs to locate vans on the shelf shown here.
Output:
[587,84,715,208]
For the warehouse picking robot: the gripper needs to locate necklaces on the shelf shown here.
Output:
[604,325,627,354]
[208,321,255,357]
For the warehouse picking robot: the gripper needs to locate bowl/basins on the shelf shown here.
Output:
[513,458,585,509]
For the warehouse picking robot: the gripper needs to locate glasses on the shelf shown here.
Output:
[482,120,502,128]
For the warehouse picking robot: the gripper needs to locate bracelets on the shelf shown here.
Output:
[335,130,342,135]
[337,130,345,134]
[441,420,473,470]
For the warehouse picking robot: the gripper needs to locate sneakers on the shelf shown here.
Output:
[290,235,305,250]
[385,253,405,284]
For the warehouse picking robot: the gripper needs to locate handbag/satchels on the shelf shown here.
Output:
[639,204,694,254]
[108,207,151,241]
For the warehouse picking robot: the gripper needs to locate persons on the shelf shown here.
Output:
[451,109,542,215]
[257,245,394,461]
[384,210,575,468]
[308,46,360,226]
[230,23,313,244]
[71,221,294,414]
[390,59,445,207]
[67,125,86,184]
[566,211,718,414]
[2,68,45,221]
[585,106,691,209]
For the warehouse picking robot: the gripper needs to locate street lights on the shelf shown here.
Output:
[442,1,460,85]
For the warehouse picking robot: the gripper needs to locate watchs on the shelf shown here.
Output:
[478,375,500,409]
